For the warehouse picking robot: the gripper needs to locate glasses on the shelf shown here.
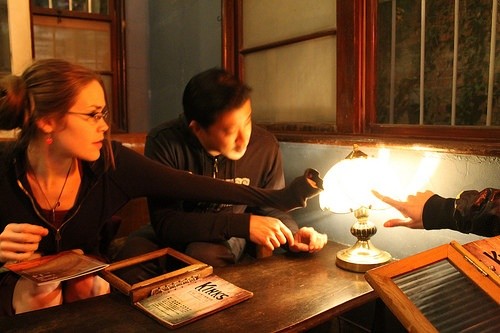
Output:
[60,108,110,122]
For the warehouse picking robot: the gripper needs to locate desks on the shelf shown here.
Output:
[0,240,399,333]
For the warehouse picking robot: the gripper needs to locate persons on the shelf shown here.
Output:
[369,187,500,238]
[0,58,324,314]
[145,68,329,254]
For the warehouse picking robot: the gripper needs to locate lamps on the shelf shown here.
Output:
[318,143,403,273]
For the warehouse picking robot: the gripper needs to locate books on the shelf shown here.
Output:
[133,275,255,330]
[4,250,112,288]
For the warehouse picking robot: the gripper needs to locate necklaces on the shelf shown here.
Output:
[25,153,76,221]
